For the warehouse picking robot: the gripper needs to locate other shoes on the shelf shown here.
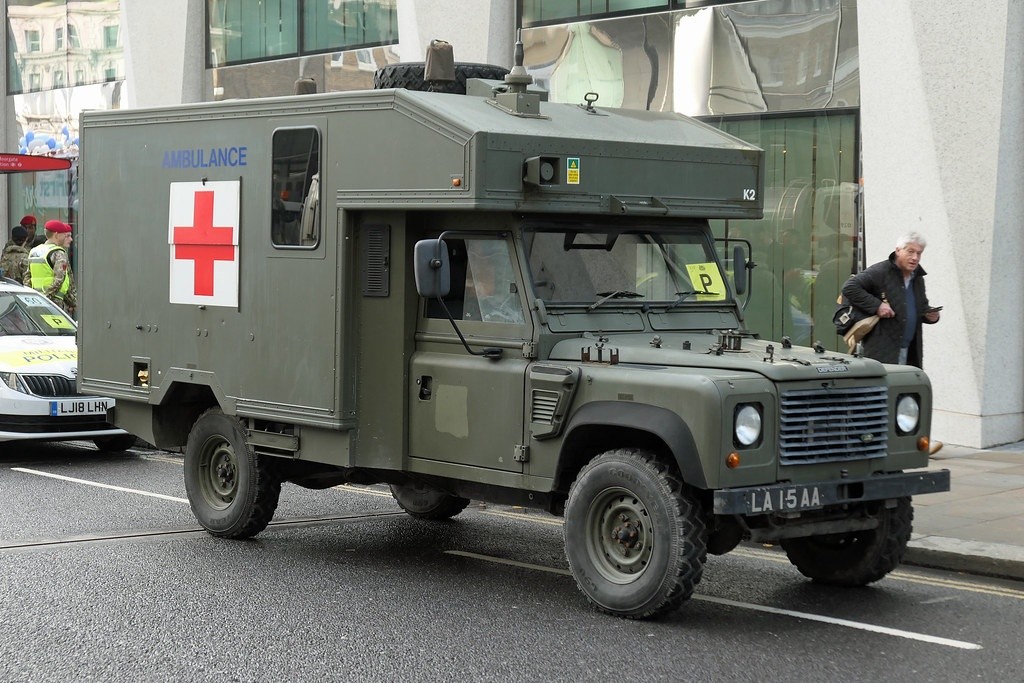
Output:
[929,441,943,455]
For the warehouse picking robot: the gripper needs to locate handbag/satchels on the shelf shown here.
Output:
[832,272,890,346]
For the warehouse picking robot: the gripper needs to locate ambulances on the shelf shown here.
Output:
[74,26,954,622]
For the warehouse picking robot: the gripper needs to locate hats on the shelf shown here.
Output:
[20,216,36,225]
[12,225,28,240]
[45,220,65,233]
[63,224,72,232]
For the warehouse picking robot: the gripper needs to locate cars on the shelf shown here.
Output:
[0,274,138,454]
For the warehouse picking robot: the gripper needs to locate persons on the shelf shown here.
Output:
[842,232,943,455]
[0,215,77,318]
